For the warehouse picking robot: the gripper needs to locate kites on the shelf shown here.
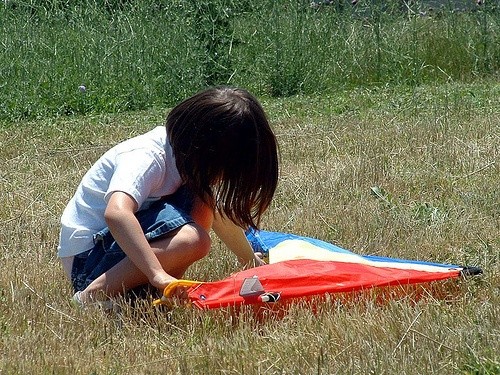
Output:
[154,225,483,309]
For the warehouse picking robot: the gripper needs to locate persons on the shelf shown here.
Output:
[54,85,279,318]
